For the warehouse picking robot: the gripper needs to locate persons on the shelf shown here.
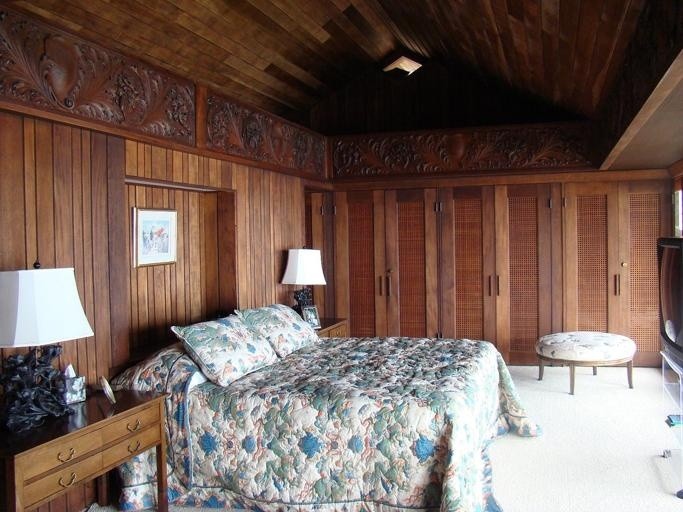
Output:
[311,313,318,325]
[306,314,312,324]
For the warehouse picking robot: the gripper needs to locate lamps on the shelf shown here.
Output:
[279,246,327,304]
[0,257,95,385]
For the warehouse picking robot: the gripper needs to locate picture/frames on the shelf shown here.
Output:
[130,206,178,269]
[300,304,322,330]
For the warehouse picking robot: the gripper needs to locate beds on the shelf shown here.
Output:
[112,330,512,510]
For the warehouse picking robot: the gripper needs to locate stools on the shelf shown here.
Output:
[533,331,638,396]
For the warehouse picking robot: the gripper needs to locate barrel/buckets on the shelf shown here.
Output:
[60,362,86,405]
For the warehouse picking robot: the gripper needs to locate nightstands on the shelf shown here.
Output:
[313,317,347,338]
[0,390,173,511]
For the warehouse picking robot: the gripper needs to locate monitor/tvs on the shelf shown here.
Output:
[656,234,683,367]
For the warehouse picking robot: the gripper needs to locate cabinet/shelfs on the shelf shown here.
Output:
[326,173,674,370]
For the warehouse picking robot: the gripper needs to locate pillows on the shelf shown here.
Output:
[169,304,321,389]
